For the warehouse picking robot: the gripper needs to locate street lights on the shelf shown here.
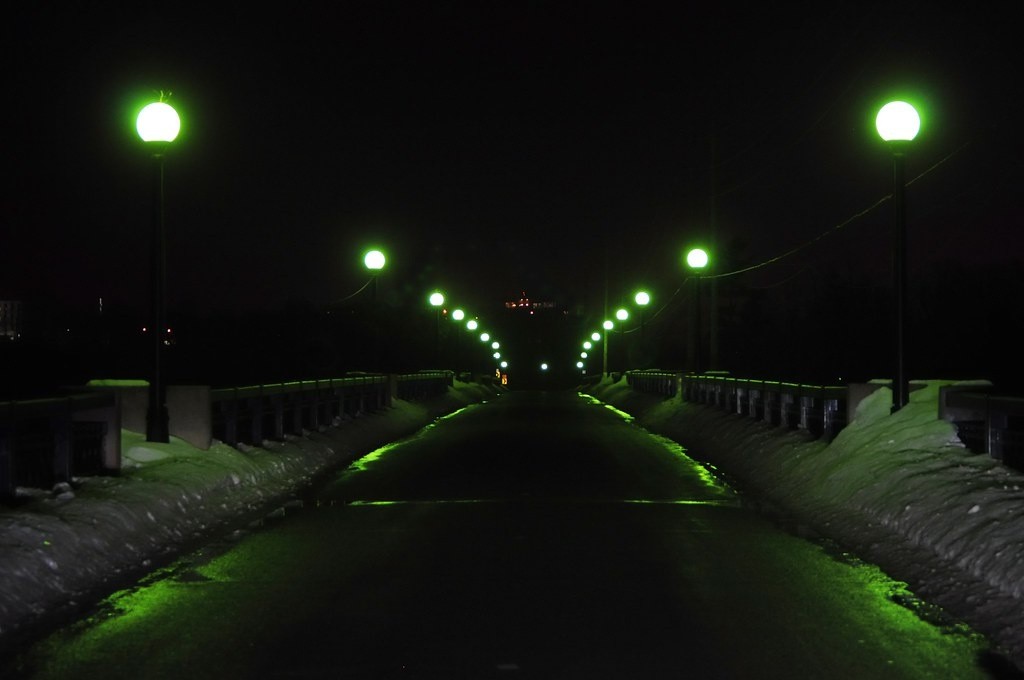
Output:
[576,331,602,374]
[684,246,711,373]
[480,332,490,374]
[615,306,630,371]
[428,290,445,371]
[451,308,465,373]
[633,288,652,372]
[492,341,508,378]
[128,94,186,436]
[466,319,479,372]
[360,247,388,377]
[602,318,614,371]
